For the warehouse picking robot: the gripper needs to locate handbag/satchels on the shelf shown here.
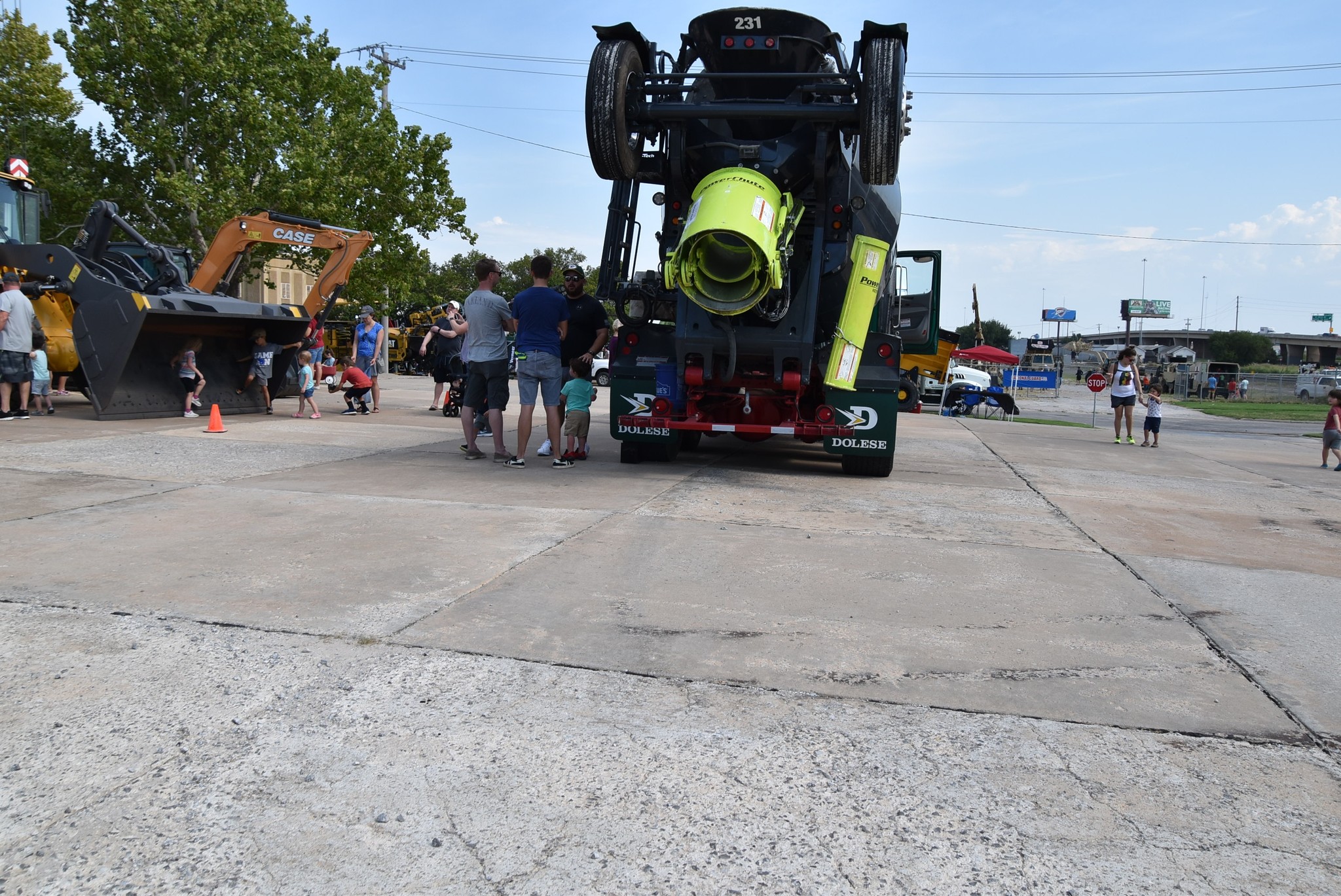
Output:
[376,355,385,374]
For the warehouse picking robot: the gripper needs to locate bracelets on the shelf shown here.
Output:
[587,350,595,358]
[1139,393,1143,394]
[449,317,455,322]
[438,328,442,333]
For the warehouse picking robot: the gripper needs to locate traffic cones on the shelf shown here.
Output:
[204,404,229,433]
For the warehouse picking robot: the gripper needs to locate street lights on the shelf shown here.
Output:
[1140,258,1148,332]
[1200,276,1207,331]
[1041,288,1046,339]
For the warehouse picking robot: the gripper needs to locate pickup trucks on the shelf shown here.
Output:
[1294,367,1341,402]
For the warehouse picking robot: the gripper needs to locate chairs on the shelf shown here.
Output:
[985,387,1006,421]
[961,386,980,418]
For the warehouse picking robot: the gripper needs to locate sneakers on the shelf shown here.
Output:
[191,396,202,406]
[266,406,273,414]
[1126,434,1135,444]
[460,427,590,469]
[184,410,199,417]
[236,385,248,395]
[1114,434,1121,444]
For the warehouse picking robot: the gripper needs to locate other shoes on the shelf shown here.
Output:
[1140,440,1150,447]
[429,405,439,410]
[1320,463,1328,468]
[292,406,382,419]
[1334,463,1341,471]
[0,387,70,420]
[1151,442,1159,447]
[313,385,320,389]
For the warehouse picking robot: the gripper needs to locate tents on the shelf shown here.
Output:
[938,345,1020,421]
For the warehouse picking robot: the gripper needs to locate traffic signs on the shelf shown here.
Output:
[1312,315,1332,321]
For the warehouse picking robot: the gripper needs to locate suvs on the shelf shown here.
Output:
[1019,352,1064,374]
[899,358,993,416]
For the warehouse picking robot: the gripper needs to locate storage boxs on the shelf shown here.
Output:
[910,401,922,413]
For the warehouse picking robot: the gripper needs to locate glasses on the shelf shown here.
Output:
[1124,354,1136,362]
[564,276,583,282]
[490,271,502,276]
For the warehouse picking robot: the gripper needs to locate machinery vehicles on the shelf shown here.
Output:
[0,170,374,422]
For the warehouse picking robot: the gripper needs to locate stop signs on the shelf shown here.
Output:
[1087,373,1107,393]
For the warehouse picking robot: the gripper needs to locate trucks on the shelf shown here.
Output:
[1153,360,1240,399]
[1259,326,1276,334]
[583,3,944,478]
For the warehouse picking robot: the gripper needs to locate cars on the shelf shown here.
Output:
[587,347,611,387]
[1318,332,1341,336]
[1229,330,1239,332]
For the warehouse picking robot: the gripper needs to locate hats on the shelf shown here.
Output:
[449,300,460,310]
[250,329,266,339]
[2,272,19,282]
[360,305,374,318]
[563,264,585,277]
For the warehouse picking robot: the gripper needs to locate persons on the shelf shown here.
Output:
[235,328,301,414]
[321,348,335,367]
[420,252,609,469]
[1207,374,1216,401]
[291,351,321,418]
[608,318,624,377]
[1227,378,1236,401]
[1002,364,1063,391]
[0,272,70,421]
[1320,389,1341,471]
[329,306,384,415]
[1236,376,1249,400]
[1143,373,1154,394]
[303,311,325,389]
[1139,383,1162,448]
[170,335,206,418]
[945,385,966,417]
[1215,367,1230,387]
[1092,367,1102,375]
[1076,366,1083,385]
[1107,346,1143,444]
